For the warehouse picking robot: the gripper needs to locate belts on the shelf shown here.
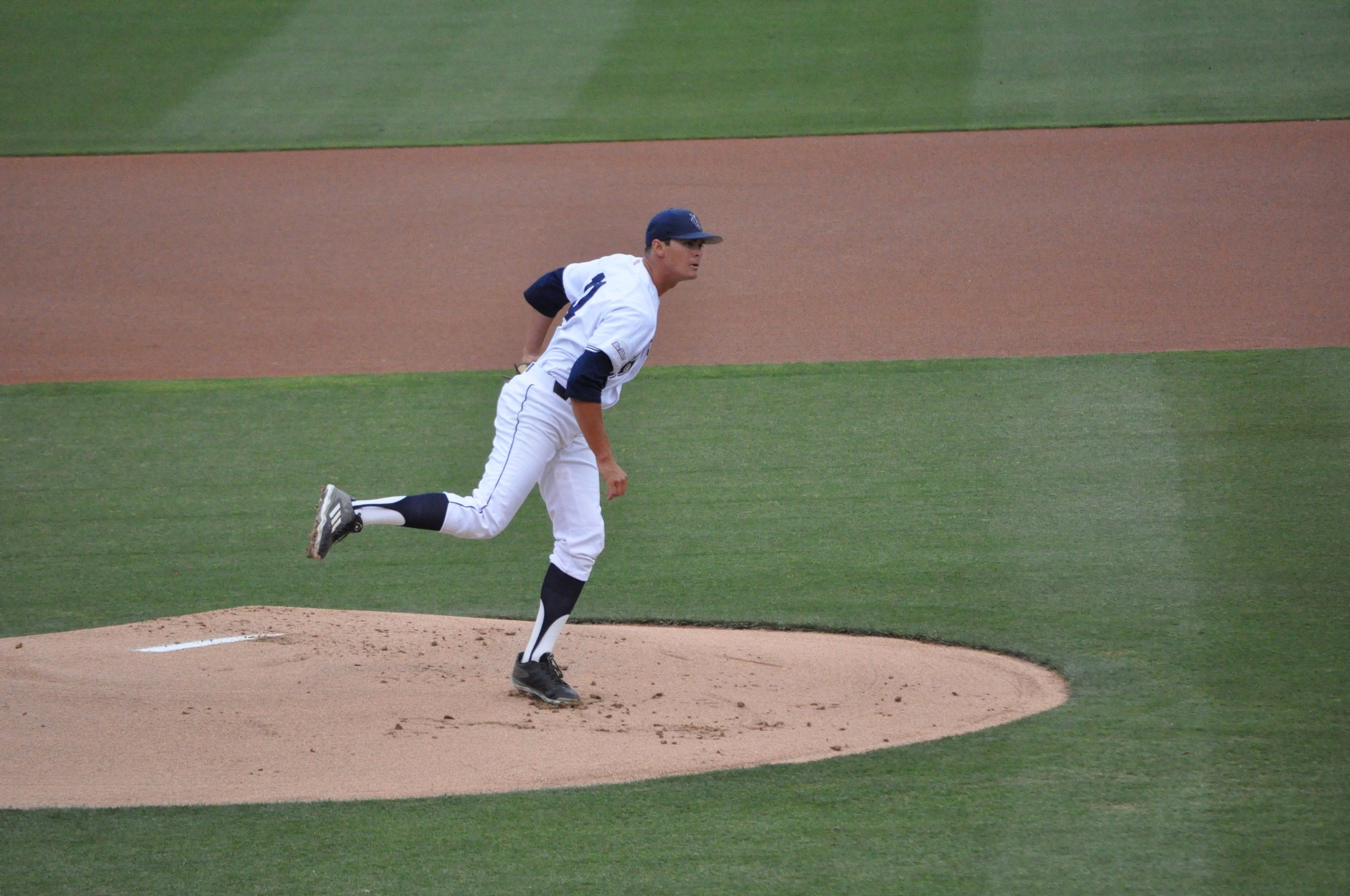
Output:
[525,363,569,401]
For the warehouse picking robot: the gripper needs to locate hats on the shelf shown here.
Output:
[646,208,723,245]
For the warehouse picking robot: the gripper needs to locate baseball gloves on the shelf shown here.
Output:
[516,364,529,375]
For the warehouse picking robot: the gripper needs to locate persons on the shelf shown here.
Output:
[307,206,725,710]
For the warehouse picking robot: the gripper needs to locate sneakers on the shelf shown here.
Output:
[511,651,580,706]
[307,484,363,561]
